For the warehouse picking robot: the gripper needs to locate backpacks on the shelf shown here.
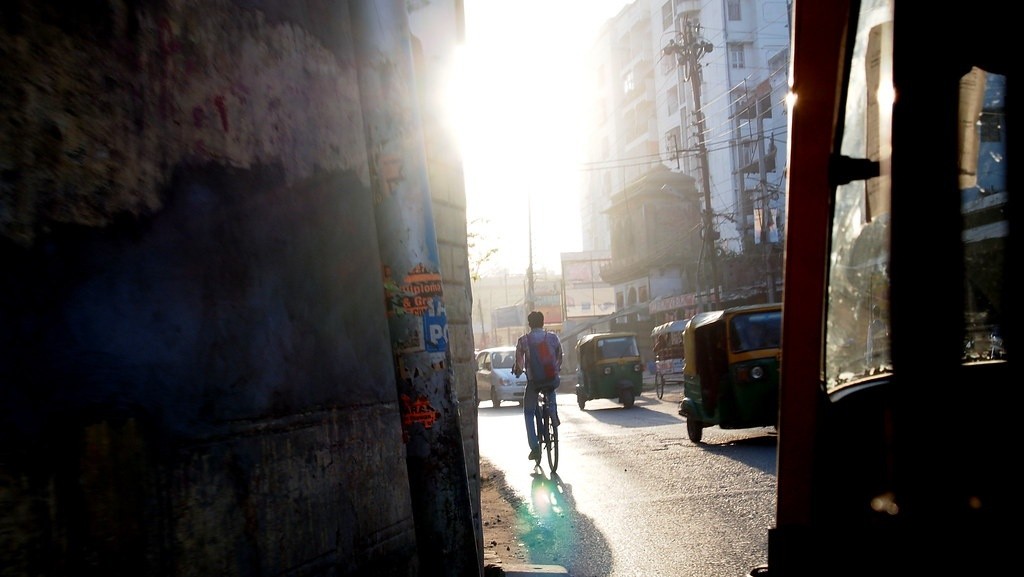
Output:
[526,332,556,382]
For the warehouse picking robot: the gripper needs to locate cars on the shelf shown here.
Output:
[475,347,528,407]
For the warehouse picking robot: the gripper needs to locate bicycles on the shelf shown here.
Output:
[510,369,563,472]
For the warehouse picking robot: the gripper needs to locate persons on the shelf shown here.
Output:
[652,334,667,351]
[511,311,563,460]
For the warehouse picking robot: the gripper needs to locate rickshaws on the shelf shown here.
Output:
[650,320,690,398]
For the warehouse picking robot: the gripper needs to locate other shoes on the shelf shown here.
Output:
[552,416,560,427]
[529,450,540,460]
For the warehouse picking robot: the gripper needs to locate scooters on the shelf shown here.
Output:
[575,332,643,410]
[677,303,782,442]
